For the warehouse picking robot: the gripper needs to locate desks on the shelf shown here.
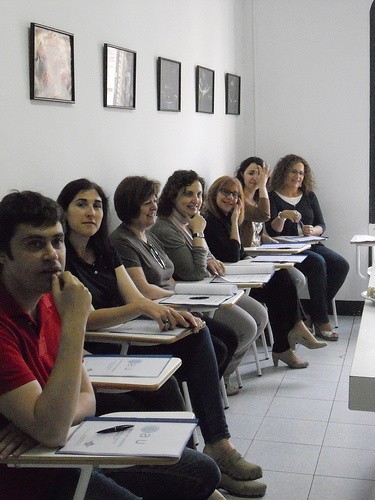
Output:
[348,275,375,412]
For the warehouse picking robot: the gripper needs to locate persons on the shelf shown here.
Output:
[265,154,350,340]
[56,179,266,498]
[197,177,328,368]
[108,176,237,383]
[0,191,221,500]
[148,170,268,395]
[236,157,305,292]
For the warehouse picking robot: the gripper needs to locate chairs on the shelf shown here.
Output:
[0,235,338,500]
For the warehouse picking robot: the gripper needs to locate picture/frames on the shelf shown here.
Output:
[103,43,137,110]
[196,65,214,114]
[225,73,241,115]
[29,23,75,104]
[157,56,181,112]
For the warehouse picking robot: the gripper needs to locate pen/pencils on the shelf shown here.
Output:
[189,297,209,299]
[299,220,304,226]
[97,425,133,434]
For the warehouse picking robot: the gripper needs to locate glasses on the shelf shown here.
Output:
[220,189,240,199]
[287,169,305,176]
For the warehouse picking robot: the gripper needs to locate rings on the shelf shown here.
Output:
[163,320,168,324]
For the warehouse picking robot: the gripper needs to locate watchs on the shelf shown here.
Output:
[192,233,204,238]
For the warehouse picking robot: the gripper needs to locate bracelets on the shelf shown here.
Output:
[277,212,286,221]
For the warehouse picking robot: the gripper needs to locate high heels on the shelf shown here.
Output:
[314,318,339,341]
[288,329,328,350]
[272,352,309,368]
[304,315,313,333]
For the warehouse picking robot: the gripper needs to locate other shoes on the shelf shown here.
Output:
[224,379,240,396]
[203,444,267,498]
